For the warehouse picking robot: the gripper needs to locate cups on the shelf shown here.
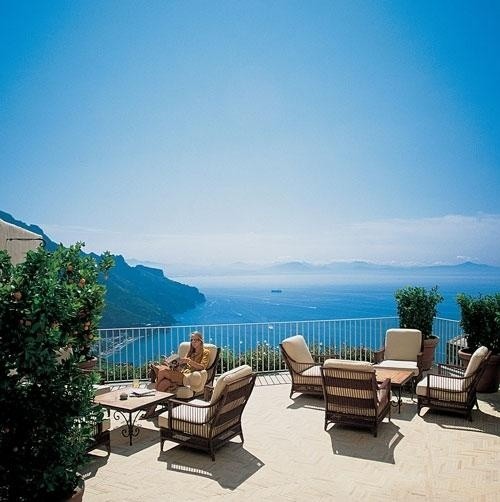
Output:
[120,393,128,400]
[133,379,140,389]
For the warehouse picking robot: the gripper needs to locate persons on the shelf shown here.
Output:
[142,331,209,421]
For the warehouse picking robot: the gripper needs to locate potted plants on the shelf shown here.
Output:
[0,240,115,502]
[66,247,115,387]
[395,283,444,371]
[456,291,500,394]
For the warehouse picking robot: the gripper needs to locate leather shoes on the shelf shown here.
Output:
[139,409,155,420]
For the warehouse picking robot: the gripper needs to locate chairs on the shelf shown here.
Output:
[278,344,338,400]
[70,400,110,466]
[320,367,391,437]
[416,351,492,422]
[374,334,424,396]
[157,371,257,461]
[153,347,221,411]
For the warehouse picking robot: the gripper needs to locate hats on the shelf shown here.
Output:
[183,370,207,392]
